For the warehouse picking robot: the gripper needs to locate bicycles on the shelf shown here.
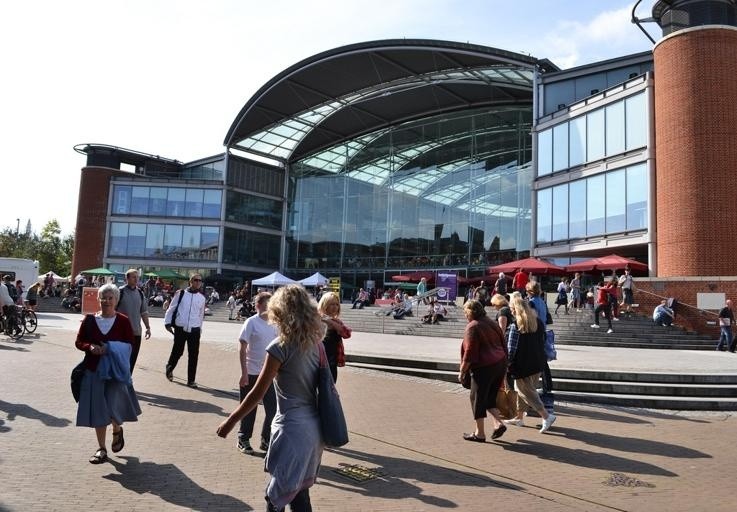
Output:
[20,309,38,334]
[0,309,27,341]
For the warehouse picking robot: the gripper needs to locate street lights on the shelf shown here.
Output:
[15,218,20,231]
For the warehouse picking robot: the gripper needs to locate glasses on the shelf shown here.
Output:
[192,278,201,281]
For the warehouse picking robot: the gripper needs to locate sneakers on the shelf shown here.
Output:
[186,382,198,388]
[607,329,613,333]
[539,415,556,434]
[504,419,525,426]
[613,318,619,322]
[260,439,269,451]
[590,324,600,328]
[577,310,582,312]
[237,438,254,454]
[166,363,174,382]
[422,318,437,324]
[716,346,727,350]
[726,347,734,352]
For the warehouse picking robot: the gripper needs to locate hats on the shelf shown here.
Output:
[506,291,522,297]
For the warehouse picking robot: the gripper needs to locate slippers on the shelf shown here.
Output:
[491,424,506,439]
[463,433,486,442]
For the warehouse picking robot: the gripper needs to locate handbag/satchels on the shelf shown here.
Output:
[338,339,345,368]
[526,393,555,416]
[496,385,517,421]
[317,343,349,448]
[71,360,87,401]
[544,330,557,361]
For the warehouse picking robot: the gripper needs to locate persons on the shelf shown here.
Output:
[70,283,142,464]
[729,335,737,353]
[716,300,736,352]
[216,284,348,512]
[318,291,352,384]
[653,300,675,327]
[236,291,281,456]
[116,268,151,376]
[165,273,206,388]
[0,274,256,337]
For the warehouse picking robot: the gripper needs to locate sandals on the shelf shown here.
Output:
[89,448,107,463]
[112,427,124,453]
